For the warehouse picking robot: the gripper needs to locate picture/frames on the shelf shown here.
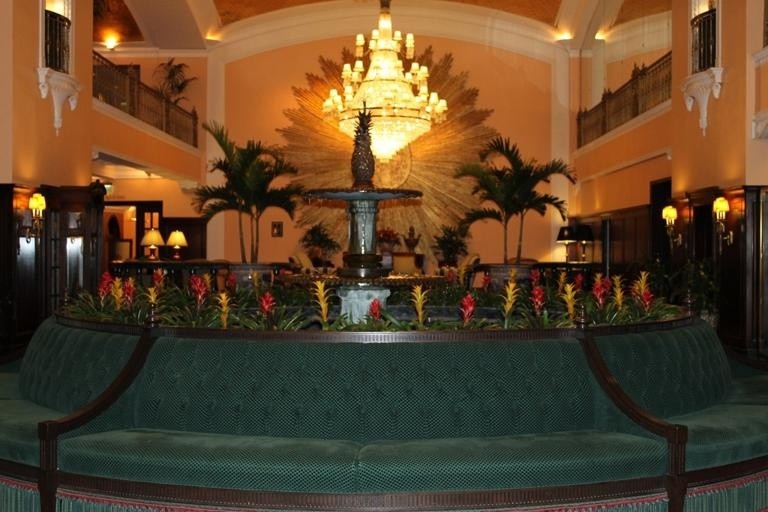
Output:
[270,220,283,237]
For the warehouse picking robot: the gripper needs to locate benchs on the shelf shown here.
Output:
[0,316,768,512]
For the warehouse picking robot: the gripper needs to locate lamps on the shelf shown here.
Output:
[25,193,46,243]
[320,0,448,166]
[661,204,683,253]
[139,228,188,262]
[709,196,734,255]
